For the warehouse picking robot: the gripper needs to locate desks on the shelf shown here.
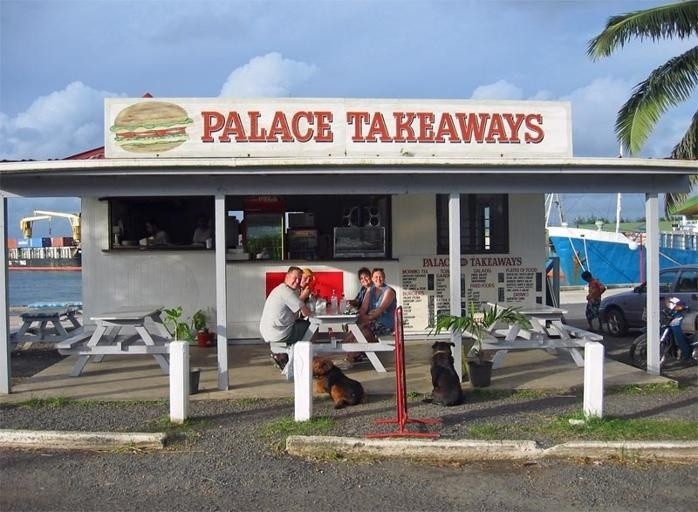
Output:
[90,304,166,348]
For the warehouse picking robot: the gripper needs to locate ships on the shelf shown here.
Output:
[8,209,82,271]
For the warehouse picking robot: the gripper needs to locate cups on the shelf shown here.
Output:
[204,238,212,248]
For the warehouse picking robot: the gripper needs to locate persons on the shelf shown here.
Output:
[191,214,212,244]
[259,266,310,345]
[145,221,169,247]
[580,270,607,332]
[297,269,315,305]
[345,267,373,310]
[341,268,397,363]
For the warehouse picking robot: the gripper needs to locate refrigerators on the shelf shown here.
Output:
[287,210,319,262]
[239,197,287,262]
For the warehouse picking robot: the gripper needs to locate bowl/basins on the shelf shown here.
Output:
[121,241,137,246]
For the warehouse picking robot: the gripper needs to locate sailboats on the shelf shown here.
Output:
[545,134,698,286]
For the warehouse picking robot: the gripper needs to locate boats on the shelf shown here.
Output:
[545,245,565,280]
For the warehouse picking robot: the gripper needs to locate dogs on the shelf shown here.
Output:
[422,340,467,408]
[312,355,367,410]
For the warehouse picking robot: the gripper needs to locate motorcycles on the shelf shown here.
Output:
[629,282,698,370]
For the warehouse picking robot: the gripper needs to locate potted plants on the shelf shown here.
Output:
[427,299,531,387]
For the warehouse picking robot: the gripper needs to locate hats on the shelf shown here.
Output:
[302,269,315,280]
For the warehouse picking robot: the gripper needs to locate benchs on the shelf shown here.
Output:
[448,300,604,379]
[271,300,395,378]
[9,304,83,365]
[55,329,173,376]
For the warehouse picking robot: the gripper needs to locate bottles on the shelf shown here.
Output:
[330,290,337,307]
[339,293,346,312]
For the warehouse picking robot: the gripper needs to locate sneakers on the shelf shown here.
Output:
[270,353,288,372]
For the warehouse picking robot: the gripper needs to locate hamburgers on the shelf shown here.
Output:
[109,100,194,153]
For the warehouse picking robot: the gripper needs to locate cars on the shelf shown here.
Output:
[598,264,698,335]
